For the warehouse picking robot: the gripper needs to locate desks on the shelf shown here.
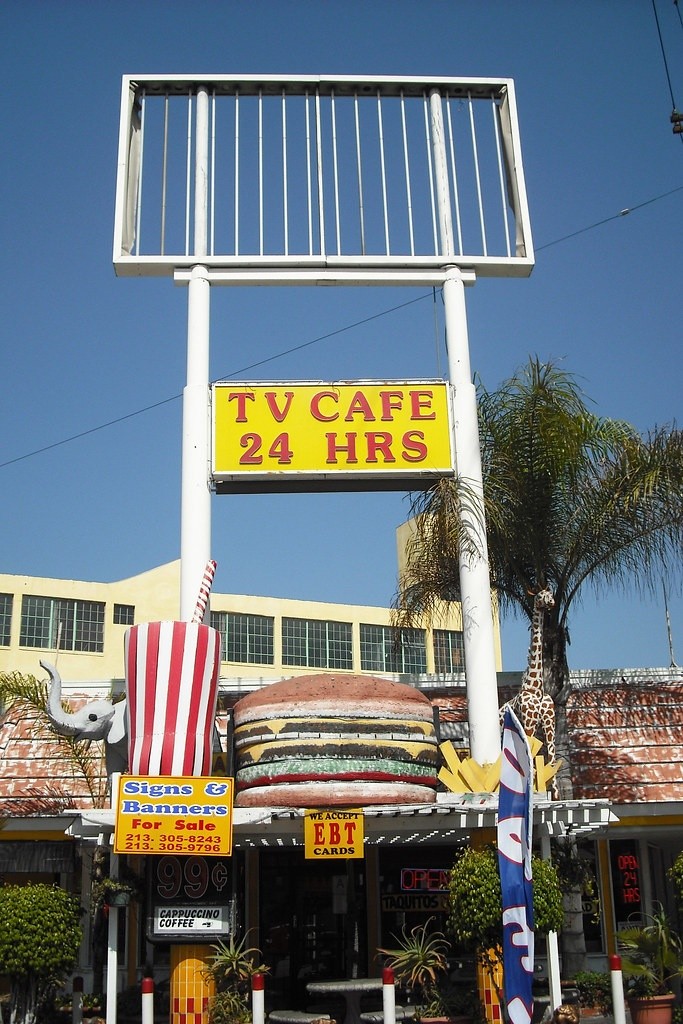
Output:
[306,977,400,1024]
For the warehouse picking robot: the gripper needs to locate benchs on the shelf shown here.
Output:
[269,1010,330,1024]
[359,1005,427,1024]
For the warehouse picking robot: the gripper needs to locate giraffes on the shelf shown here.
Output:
[498,581,560,802]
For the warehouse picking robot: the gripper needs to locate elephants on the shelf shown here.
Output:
[37,658,127,774]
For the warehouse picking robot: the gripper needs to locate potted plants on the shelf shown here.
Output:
[87,864,145,918]
[373,912,474,1024]
[612,900,683,1024]
